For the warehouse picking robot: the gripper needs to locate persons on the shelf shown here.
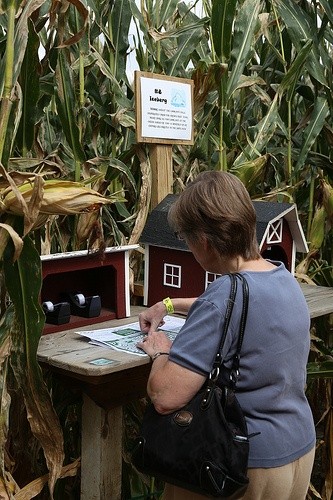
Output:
[136,169,318,500]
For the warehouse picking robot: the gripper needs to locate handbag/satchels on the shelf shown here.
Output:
[130,272,251,499]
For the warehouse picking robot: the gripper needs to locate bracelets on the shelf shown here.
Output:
[163,296,175,315]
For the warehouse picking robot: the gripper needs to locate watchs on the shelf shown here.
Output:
[151,351,168,360]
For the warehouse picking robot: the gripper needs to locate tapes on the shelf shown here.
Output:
[42,301,54,311]
[74,294,86,305]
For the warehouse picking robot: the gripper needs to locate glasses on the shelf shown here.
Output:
[174,231,184,241]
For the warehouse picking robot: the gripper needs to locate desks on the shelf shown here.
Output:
[34,280,333,500]
